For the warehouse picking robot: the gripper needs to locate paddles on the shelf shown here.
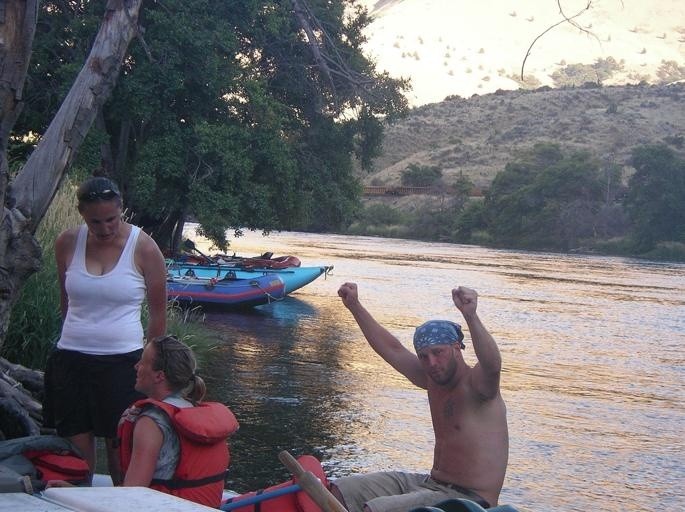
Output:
[185,239,212,262]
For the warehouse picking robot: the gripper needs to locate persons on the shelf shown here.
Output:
[327,281,510,512]
[115,334,209,491]
[46,178,167,490]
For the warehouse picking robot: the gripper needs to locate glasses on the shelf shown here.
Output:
[78,189,117,202]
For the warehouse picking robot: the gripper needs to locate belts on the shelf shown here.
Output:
[430,476,491,510]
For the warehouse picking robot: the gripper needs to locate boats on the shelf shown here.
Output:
[165,253,334,310]
